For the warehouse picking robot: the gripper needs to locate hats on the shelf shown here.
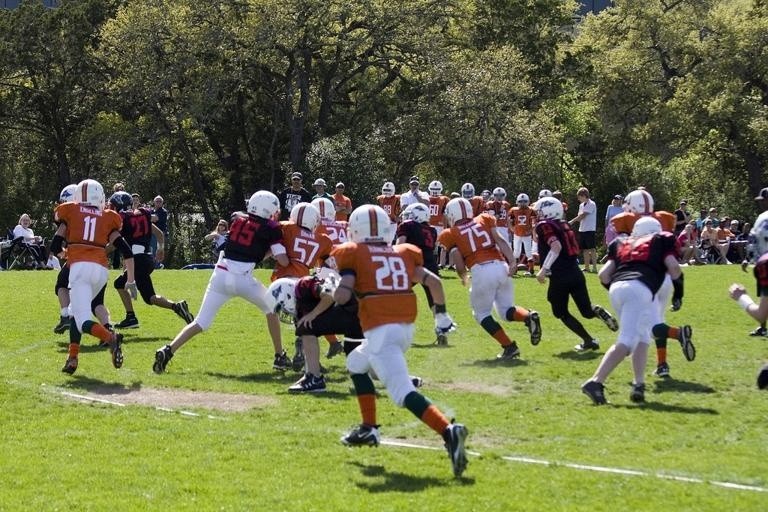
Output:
[680,188,768,228]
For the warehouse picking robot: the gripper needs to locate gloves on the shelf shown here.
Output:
[123,281,137,300]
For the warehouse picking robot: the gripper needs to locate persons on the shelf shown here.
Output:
[330,203,470,478]
[13,214,53,270]
[152,174,355,394]
[728,187,768,390]
[374,175,696,405]
[675,201,749,266]
[47,178,194,373]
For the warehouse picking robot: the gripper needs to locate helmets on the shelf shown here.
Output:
[263,277,297,323]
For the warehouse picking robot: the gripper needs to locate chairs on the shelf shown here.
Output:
[698,239,728,263]
